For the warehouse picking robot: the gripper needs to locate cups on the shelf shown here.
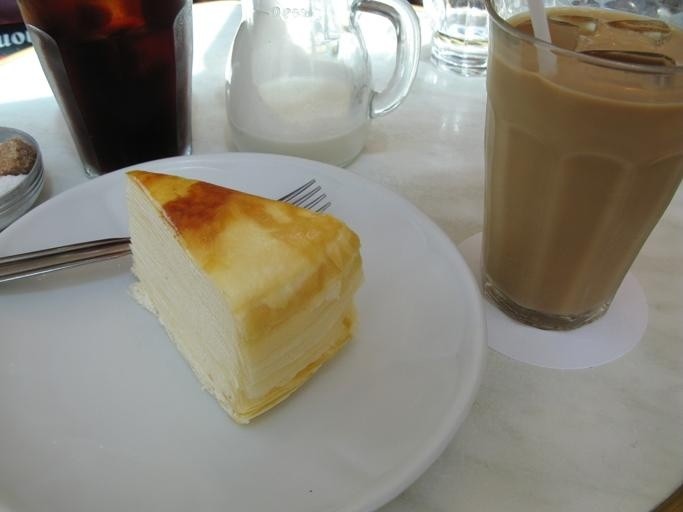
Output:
[472,1,683,338]
[219,0,424,179]
[425,0,495,80]
[16,0,198,182]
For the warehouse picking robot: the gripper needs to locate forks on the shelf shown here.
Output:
[1,173,333,288]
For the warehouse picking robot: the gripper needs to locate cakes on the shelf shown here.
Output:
[125,171,366,425]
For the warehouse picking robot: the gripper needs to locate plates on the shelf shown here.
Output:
[0,147,490,511]
[0,125,50,235]
